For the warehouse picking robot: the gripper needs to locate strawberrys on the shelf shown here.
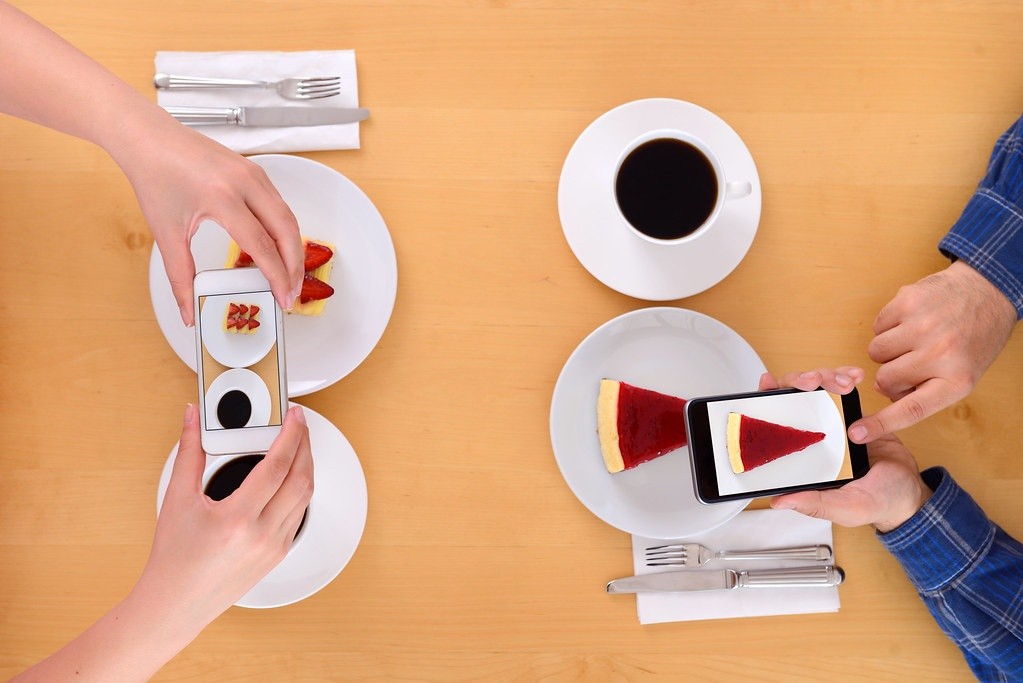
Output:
[233,241,335,304]
[226,303,260,330]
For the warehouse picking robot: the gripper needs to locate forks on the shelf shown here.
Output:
[645,544,832,568]
[153,73,341,100]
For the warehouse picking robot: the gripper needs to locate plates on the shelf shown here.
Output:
[149,154,398,401]
[157,402,367,609]
[707,390,845,496]
[205,369,271,430]
[555,97,762,302]
[549,307,770,541]
[201,292,276,369]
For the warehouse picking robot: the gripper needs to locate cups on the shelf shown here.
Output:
[214,387,253,429]
[202,452,311,559]
[611,129,726,247]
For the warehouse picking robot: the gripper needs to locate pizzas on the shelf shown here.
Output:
[726,412,826,474]
[598,379,690,474]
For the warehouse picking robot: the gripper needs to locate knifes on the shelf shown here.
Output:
[604,565,846,594]
[161,106,370,127]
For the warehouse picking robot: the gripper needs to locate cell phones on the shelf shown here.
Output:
[193,267,292,456]
[683,384,870,506]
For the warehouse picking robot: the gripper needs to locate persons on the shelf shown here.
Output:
[760,116,1023,681]
[0,0,315,682]
